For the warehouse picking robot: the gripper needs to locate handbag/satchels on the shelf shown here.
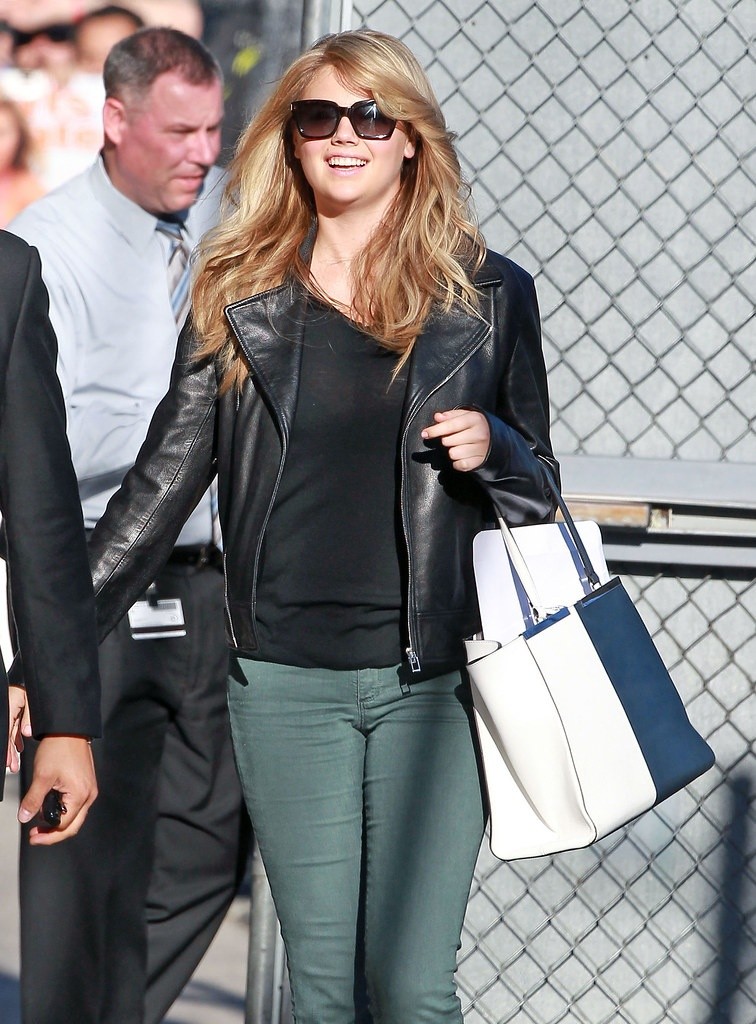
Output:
[461,454,715,862]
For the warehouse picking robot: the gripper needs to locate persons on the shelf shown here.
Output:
[6,27,562,1024]
[0,5,144,229]
[0,28,253,1024]
[0,230,105,848]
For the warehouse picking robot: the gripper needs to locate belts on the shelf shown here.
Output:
[164,544,222,567]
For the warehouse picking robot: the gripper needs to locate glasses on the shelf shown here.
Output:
[291,99,397,140]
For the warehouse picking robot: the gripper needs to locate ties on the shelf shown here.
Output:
[154,215,224,554]
